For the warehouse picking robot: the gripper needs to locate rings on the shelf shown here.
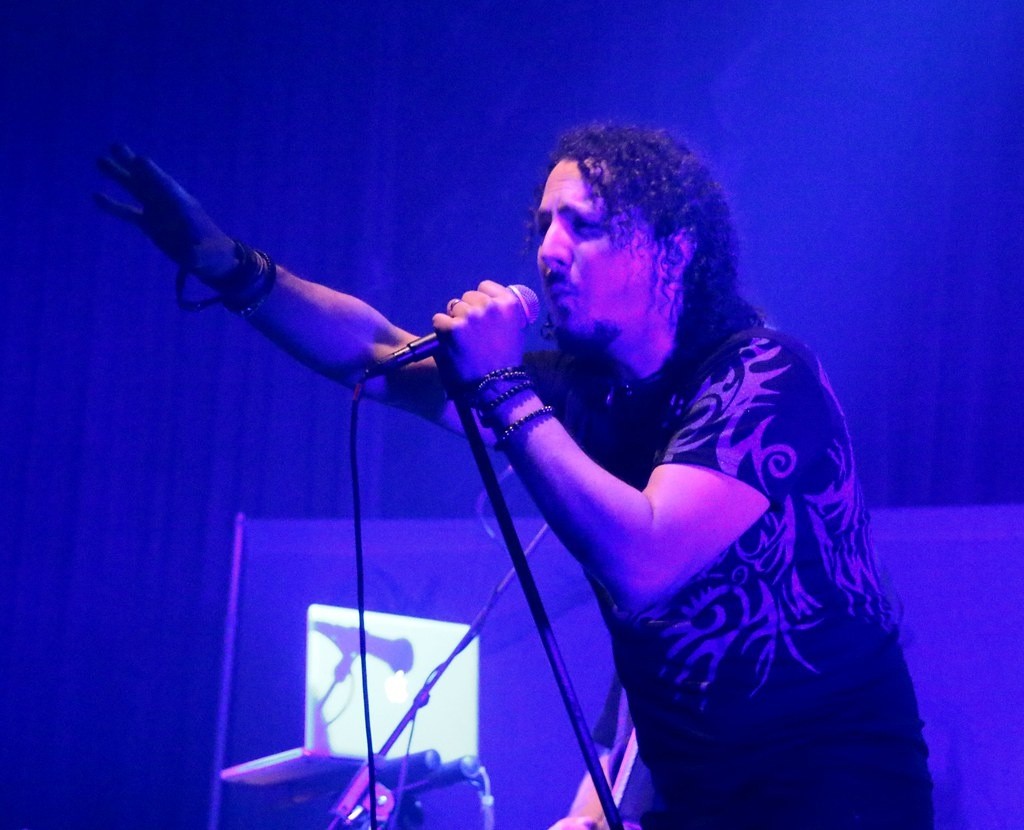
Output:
[450,299,461,317]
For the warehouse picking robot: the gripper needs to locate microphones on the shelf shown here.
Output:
[363,284,540,382]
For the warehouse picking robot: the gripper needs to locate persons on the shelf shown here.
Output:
[549,753,612,830]
[88,119,936,830]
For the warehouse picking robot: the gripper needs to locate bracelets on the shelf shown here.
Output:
[495,406,555,451]
[175,236,276,318]
[467,366,536,427]
